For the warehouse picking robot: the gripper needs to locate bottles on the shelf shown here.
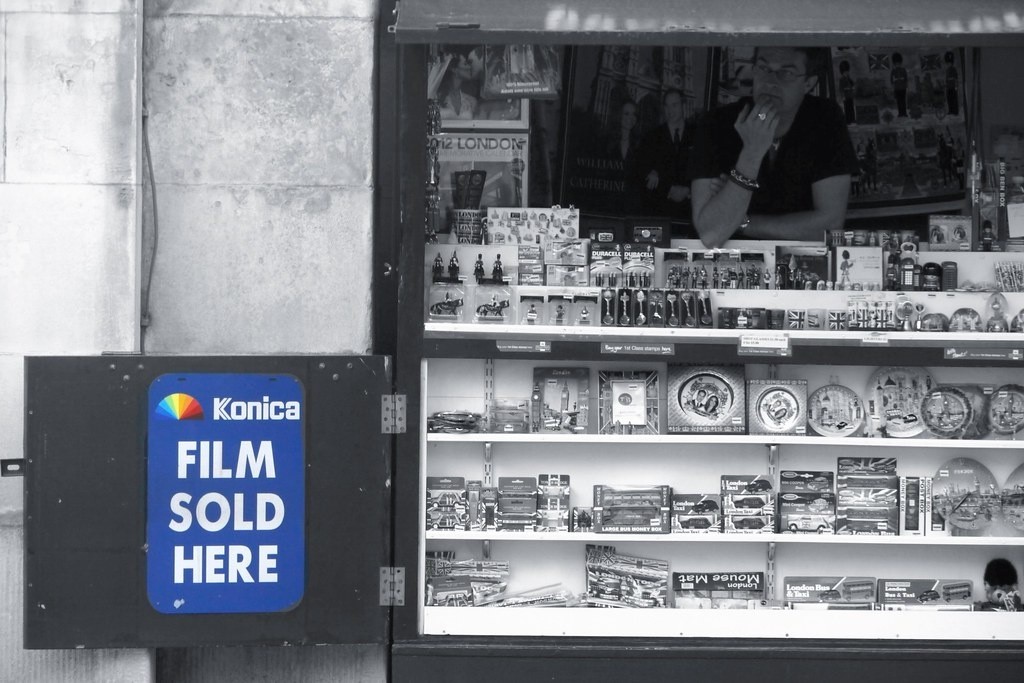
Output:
[847,300,896,328]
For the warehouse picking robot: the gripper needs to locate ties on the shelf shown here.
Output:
[697,403,699,407]
[674,128,681,153]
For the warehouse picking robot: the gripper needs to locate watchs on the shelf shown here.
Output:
[683,45,861,251]
[736,213,750,237]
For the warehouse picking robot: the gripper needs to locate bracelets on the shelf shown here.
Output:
[727,168,759,193]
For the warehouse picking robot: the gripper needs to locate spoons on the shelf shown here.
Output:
[666,293,678,327]
[634,290,647,325]
[681,291,696,327]
[650,290,662,325]
[697,290,713,324]
[603,290,613,326]
[619,290,630,326]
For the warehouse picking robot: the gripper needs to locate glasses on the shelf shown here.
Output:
[750,65,812,84]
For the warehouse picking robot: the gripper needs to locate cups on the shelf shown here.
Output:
[766,310,786,330]
[807,308,826,330]
[748,307,766,328]
[788,309,805,330]
[737,308,750,328]
[448,208,483,246]
[829,310,847,331]
[718,307,739,329]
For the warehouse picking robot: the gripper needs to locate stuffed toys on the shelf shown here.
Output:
[974,558,1024,611]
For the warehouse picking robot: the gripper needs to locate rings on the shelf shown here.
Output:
[757,113,766,122]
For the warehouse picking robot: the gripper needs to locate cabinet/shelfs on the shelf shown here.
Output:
[412,41,1024,642]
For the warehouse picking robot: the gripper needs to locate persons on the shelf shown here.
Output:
[663,263,801,289]
[977,220,995,252]
[432,250,504,281]
[836,49,967,196]
[432,45,694,216]
[690,388,718,416]
